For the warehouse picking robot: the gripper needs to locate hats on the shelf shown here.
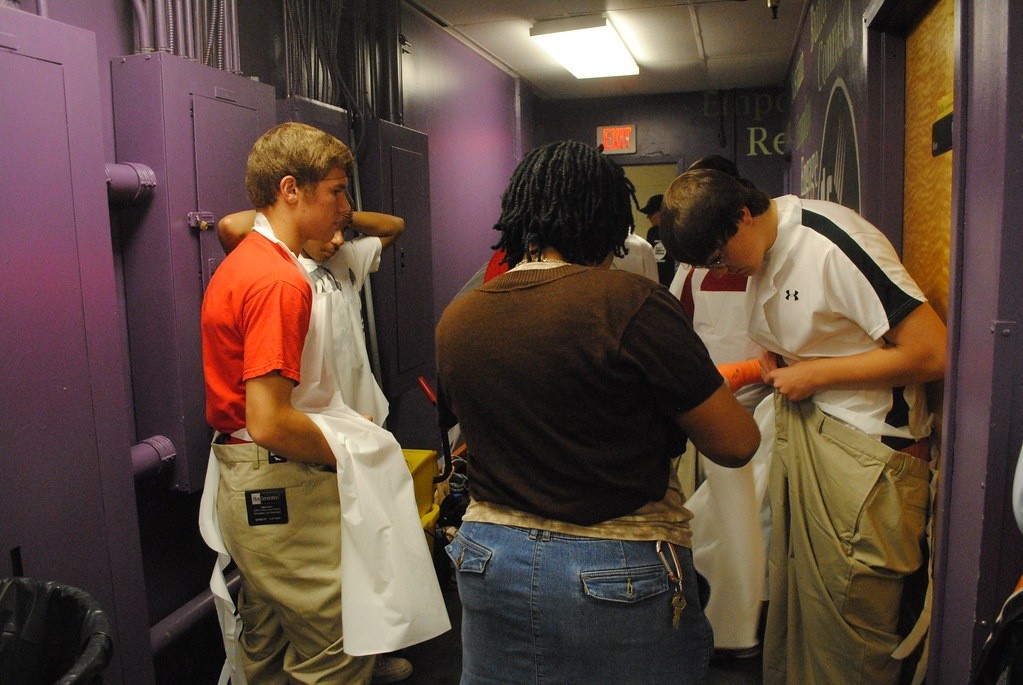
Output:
[639,194,663,214]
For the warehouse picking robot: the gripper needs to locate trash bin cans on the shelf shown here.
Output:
[1,576,112,685]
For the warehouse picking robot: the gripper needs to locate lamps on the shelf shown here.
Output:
[529,13,640,80]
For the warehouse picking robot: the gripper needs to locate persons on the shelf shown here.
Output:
[432,137,765,684]
[455,207,672,299]
[654,168,948,685]
[663,151,775,664]
[198,120,393,685]
[216,204,417,685]
[639,188,675,291]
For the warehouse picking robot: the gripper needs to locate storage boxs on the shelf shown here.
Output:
[400,449,438,563]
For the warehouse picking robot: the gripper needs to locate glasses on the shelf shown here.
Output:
[692,223,730,269]
